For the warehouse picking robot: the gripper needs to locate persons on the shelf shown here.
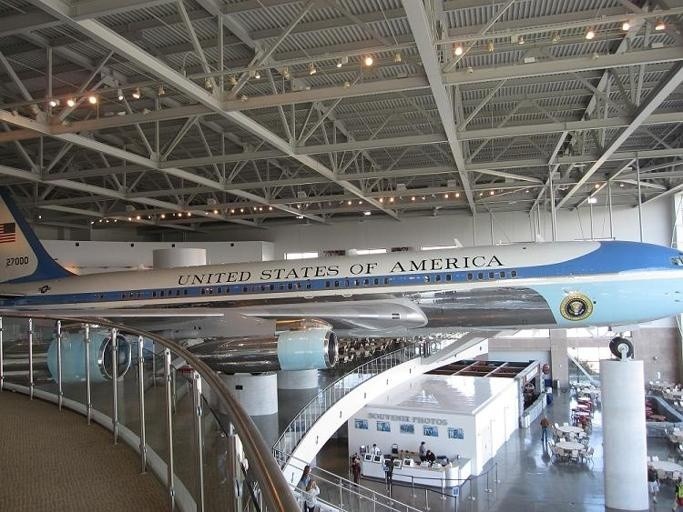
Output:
[670,475,683,512]
[384,457,395,490]
[300,465,311,488]
[418,441,425,461]
[539,415,549,442]
[648,463,660,504]
[424,449,434,462]
[368,443,380,455]
[351,456,361,486]
[303,479,320,511]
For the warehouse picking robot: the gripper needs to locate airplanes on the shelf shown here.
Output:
[0,184,683,381]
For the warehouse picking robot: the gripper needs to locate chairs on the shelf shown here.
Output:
[549,382,597,466]
[645,378,683,487]
